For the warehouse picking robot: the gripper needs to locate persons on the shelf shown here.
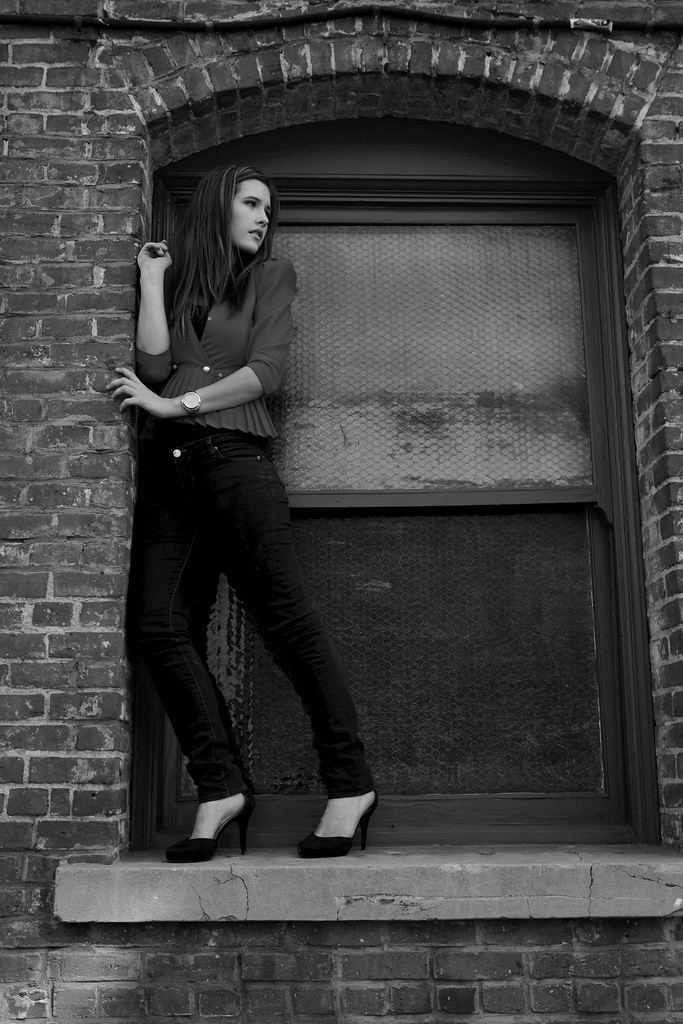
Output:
[106,164,378,862]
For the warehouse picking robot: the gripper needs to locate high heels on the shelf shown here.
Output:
[297,789,378,858]
[166,790,255,864]
